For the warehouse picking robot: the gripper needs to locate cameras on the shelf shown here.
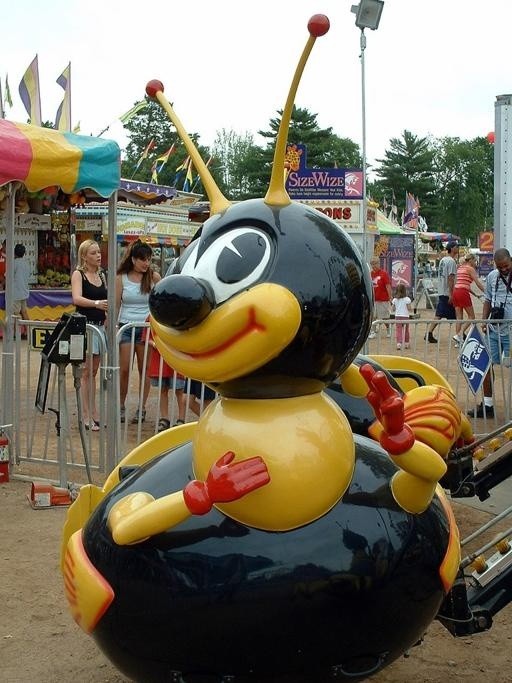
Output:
[490,307,504,325]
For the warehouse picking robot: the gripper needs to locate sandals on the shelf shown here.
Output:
[78,418,105,431]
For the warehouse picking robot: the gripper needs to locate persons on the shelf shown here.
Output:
[368,241,512,419]
[13,244,32,340]
[71,239,215,433]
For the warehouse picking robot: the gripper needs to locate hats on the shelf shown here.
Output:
[446,241,461,249]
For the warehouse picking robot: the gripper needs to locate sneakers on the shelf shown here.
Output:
[453,333,465,347]
[465,402,495,419]
[171,418,185,426]
[423,332,438,344]
[131,407,147,424]
[120,406,126,423]
[368,331,376,338]
[156,417,170,432]
[386,324,391,337]
[396,341,410,350]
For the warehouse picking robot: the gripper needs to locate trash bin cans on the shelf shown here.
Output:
[426,287,438,309]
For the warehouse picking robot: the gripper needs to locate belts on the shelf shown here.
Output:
[86,320,104,325]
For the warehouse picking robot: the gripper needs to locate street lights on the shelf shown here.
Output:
[348,0,389,338]
